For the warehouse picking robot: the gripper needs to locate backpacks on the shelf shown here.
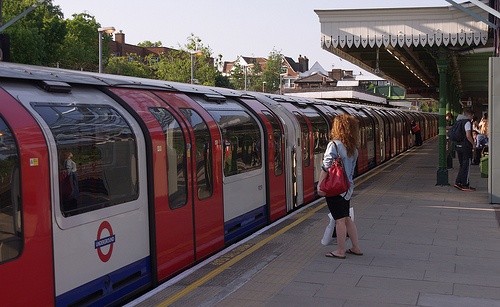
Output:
[447,119,471,143]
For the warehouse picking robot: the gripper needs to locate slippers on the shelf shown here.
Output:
[345,248,363,255]
[326,252,346,259]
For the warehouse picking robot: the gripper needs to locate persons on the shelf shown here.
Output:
[470,113,488,165]
[321,114,363,259]
[63,152,77,203]
[454,106,476,191]
[411,120,422,146]
[445,112,453,169]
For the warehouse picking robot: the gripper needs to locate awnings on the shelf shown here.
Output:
[313,1,494,89]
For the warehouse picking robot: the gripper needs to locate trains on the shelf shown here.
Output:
[0,61,436,307]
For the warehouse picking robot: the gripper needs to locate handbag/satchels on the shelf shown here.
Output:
[319,141,350,197]
[321,206,355,246]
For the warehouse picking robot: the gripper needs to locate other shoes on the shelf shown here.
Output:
[454,184,462,190]
[461,186,475,191]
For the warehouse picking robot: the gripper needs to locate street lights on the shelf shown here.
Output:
[279,72,288,95]
[262,82,266,92]
[190,49,204,84]
[244,63,254,90]
[98,26,116,75]
